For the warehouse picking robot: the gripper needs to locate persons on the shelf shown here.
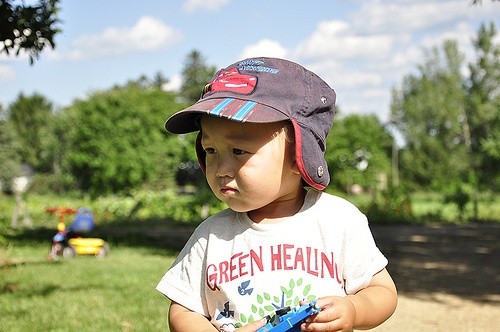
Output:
[153,56,399,332]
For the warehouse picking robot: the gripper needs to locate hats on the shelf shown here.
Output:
[164,57,335,190]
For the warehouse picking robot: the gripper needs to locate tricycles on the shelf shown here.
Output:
[45,205,109,259]
